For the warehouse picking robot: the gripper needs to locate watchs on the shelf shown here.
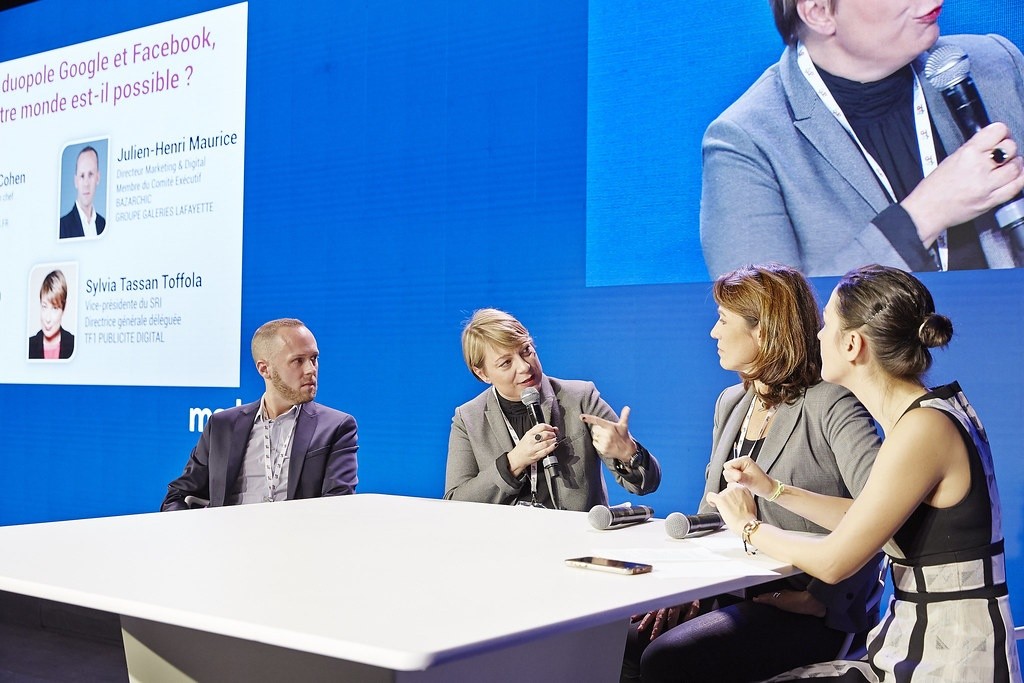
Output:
[618,444,644,470]
[743,519,763,556]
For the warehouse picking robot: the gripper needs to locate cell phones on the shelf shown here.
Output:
[563,557,652,575]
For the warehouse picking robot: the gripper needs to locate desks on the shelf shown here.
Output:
[0,493,831,683]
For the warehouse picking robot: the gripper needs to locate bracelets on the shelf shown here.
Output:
[765,479,785,502]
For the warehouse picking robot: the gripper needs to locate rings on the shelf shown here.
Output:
[989,147,1009,165]
[535,433,542,442]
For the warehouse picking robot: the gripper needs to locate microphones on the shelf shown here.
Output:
[520,387,559,478]
[587,504,655,530]
[926,44,1024,252]
[665,512,725,539]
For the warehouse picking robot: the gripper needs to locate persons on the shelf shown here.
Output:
[61,147,105,238]
[442,308,661,512]
[159,316,360,513]
[699,0,1024,281]
[620,263,891,683]
[29,270,74,358]
[709,263,1021,683]
